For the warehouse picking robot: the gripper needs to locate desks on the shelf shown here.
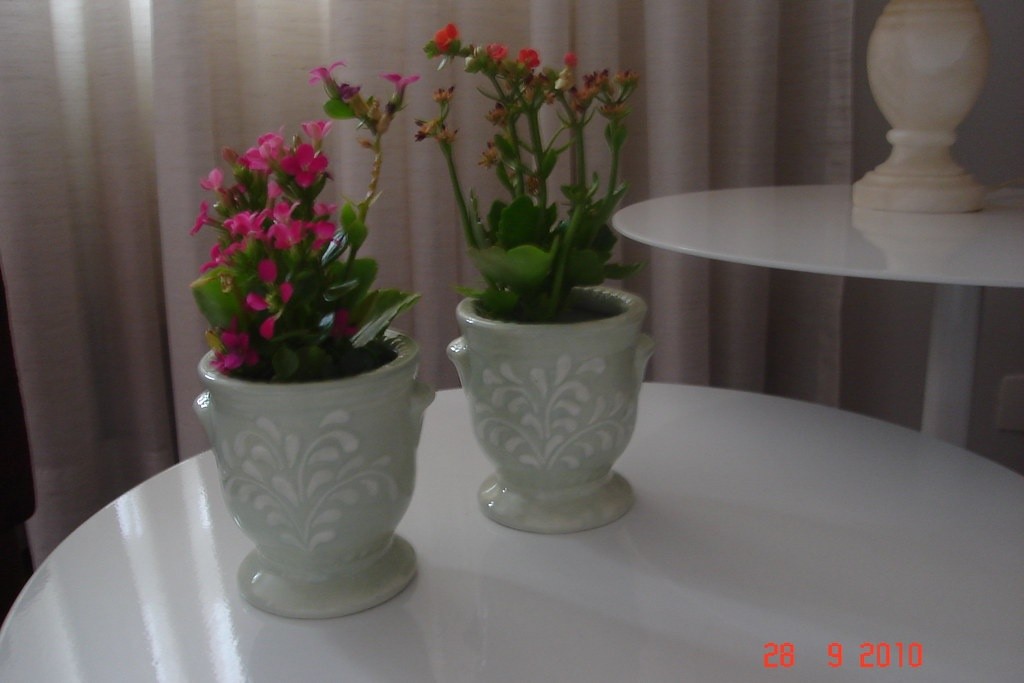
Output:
[611,182,1024,452]
[0,383,1024,683]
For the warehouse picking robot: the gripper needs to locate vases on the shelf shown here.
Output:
[194,330,429,621]
[447,284,660,533]
[848,0,986,215]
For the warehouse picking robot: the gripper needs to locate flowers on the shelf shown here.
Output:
[410,21,646,326]
[189,62,421,386]
